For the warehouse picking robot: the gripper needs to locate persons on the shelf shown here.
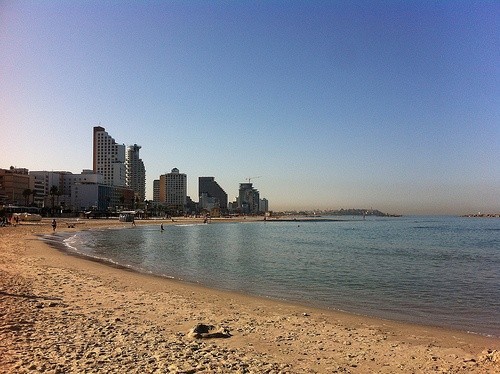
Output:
[132,220,136,227]
[0,215,19,227]
[66,223,75,228]
[51,219,57,232]
[160,222,164,232]
[264,217,266,222]
